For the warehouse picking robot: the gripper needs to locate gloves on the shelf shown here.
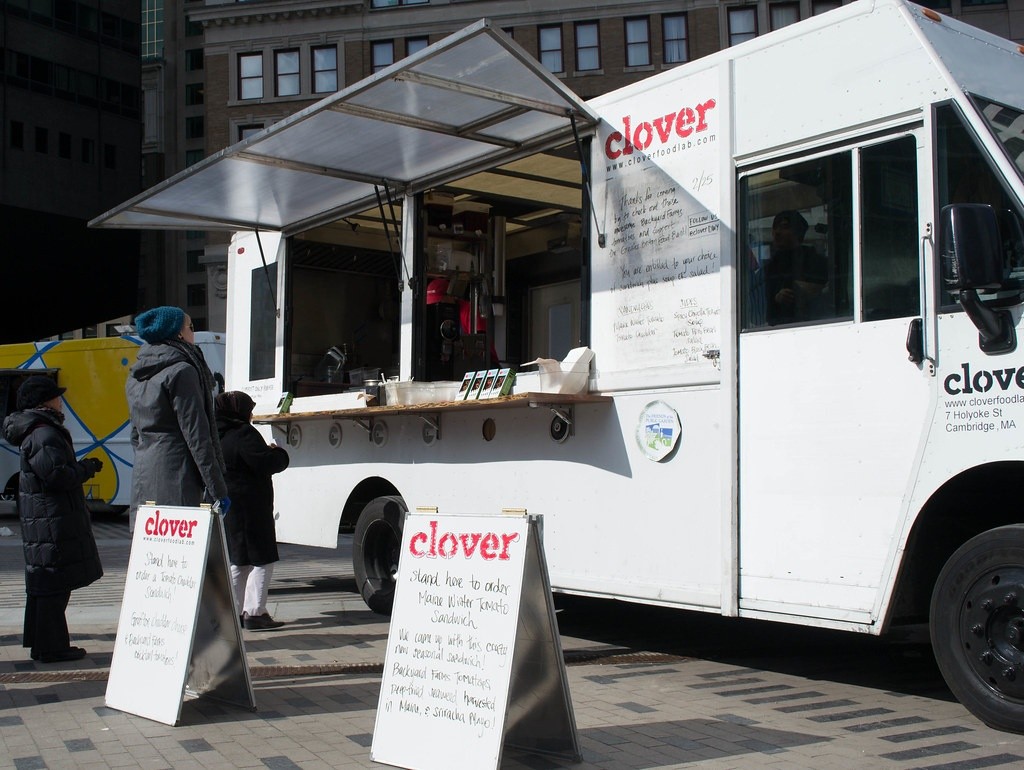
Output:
[216,497,231,518]
[83,458,104,480]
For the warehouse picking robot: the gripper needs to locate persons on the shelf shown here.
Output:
[214,390,290,630]
[765,209,827,326]
[124,306,230,533]
[2,377,104,662]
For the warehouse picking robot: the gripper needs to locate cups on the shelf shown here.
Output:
[364,380,381,386]
[366,386,380,406]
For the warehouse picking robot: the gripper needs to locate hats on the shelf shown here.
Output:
[426,277,455,306]
[134,306,184,344]
[17,376,67,410]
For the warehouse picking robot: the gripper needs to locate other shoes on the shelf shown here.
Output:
[238,611,286,632]
[30,646,87,663]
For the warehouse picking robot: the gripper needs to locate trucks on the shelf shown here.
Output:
[86,1,1024,737]
[0,333,226,519]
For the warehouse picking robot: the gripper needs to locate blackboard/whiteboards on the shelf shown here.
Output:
[367,516,531,770]
[101,502,213,726]
[591,62,733,392]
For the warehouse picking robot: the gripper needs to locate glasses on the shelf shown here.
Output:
[185,323,195,332]
